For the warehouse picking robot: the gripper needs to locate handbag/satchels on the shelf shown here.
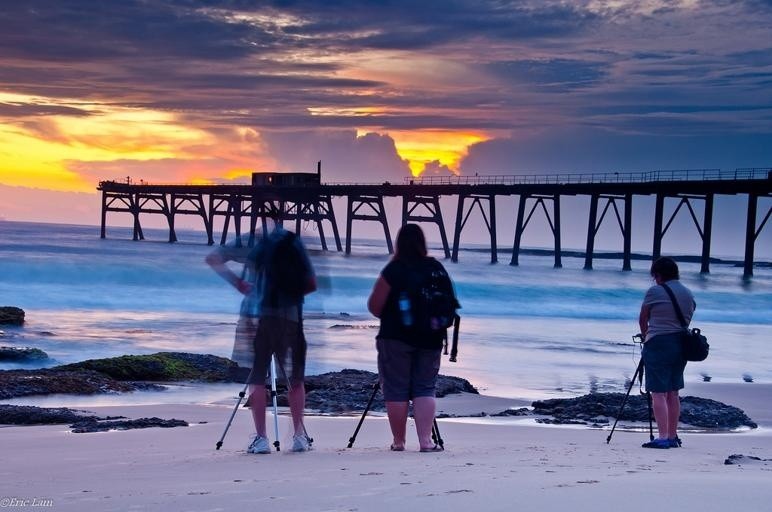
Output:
[679,328,709,362]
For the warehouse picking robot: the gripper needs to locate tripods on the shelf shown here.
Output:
[605,351,658,444]
[215,350,316,451]
[345,365,444,450]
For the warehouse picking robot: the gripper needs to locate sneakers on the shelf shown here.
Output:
[246,436,270,453]
[289,434,313,452]
[642,438,679,448]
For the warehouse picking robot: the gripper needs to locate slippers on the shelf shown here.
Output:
[420,444,443,452]
[391,444,404,450]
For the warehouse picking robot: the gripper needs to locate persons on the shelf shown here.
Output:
[636,256,697,449]
[203,203,320,454]
[366,222,462,453]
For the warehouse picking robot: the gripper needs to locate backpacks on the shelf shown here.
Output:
[408,254,456,331]
[253,231,304,310]
